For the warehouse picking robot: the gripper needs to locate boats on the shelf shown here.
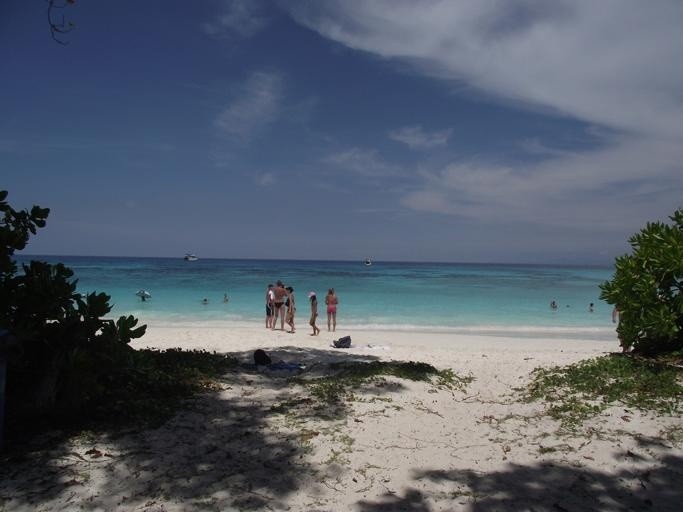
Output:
[364,260,372,266]
[188,255,198,261]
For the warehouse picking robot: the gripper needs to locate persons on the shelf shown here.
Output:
[611,306,618,324]
[325,287,338,332]
[589,303,594,312]
[550,299,558,309]
[308,291,320,336]
[202,299,209,304]
[224,293,229,303]
[265,280,296,333]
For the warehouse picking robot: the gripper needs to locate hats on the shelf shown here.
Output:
[308,292,316,297]
[286,287,294,291]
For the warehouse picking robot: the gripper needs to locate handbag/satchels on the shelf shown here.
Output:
[334,336,352,349]
